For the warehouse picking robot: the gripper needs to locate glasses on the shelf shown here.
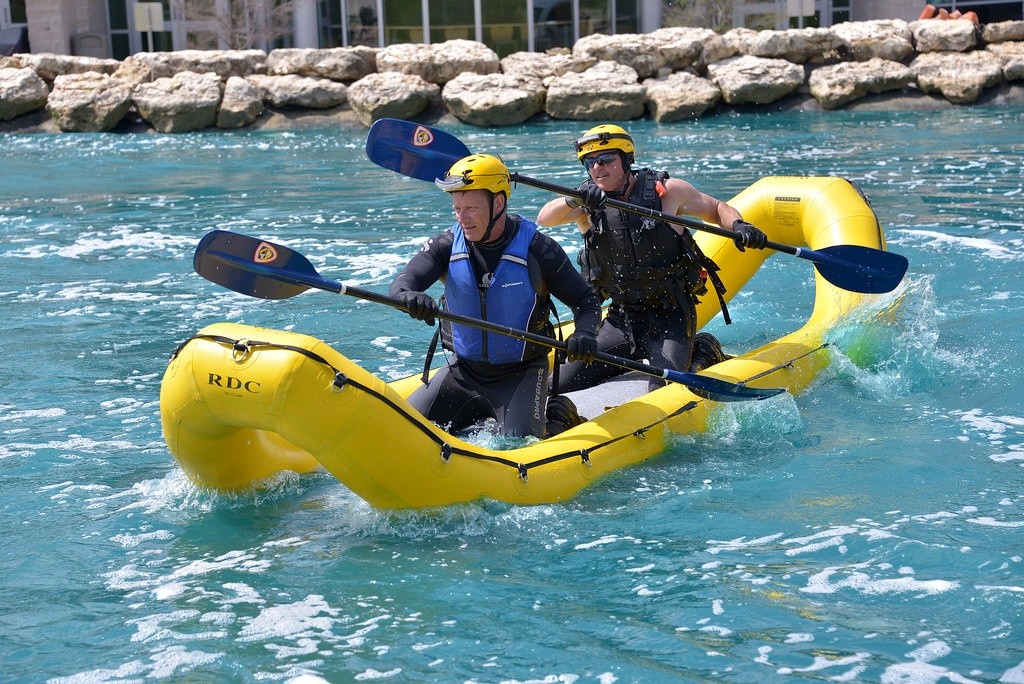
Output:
[584,151,620,168]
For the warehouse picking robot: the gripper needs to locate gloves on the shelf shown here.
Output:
[732,218,767,252]
[396,290,439,326]
[558,327,597,364]
[565,184,608,212]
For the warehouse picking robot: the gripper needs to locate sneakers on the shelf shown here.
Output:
[546,395,588,432]
[692,333,734,370]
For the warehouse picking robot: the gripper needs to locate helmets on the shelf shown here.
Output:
[435,154,511,200]
[574,125,635,165]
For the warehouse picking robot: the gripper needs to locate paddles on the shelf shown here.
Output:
[362,118,911,297]
[190,229,786,402]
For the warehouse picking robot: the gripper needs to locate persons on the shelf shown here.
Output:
[389,152,601,436]
[537,123,767,396]
